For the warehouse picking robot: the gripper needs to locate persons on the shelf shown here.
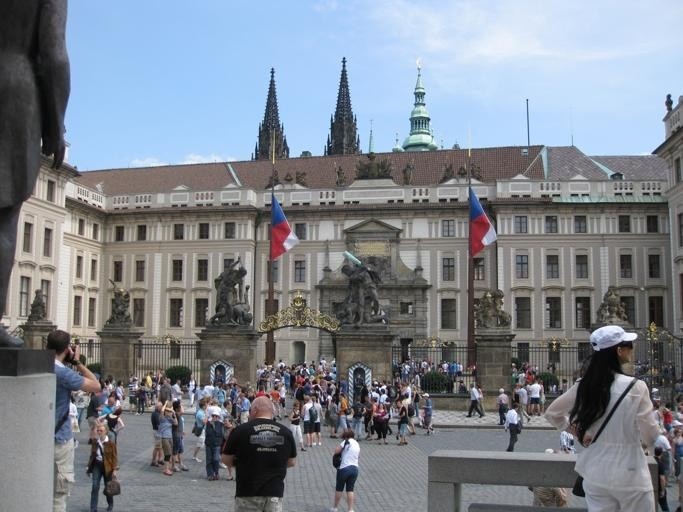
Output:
[496,359,683,512]
[333,430,360,511]
[45,331,101,510]
[340,261,384,323]
[212,254,255,323]
[0,1,70,347]
[543,325,661,512]
[86,424,118,511]
[69,358,486,479]
[220,396,297,512]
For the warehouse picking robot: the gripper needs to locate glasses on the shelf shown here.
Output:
[619,341,635,349]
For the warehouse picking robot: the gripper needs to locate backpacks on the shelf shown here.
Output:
[309,402,318,422]
[353,403,364,419]
[408,402,415,417]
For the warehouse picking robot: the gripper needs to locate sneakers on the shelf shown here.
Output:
[301,441,323,452]
[497,418,506,425]
[466,412,487,418]
[128,402,156,417]
[321,413,435,447]
[518,412,542,425]
[150,457,233,482]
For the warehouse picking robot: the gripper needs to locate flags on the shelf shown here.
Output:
[271,195,299,262]
[468,187,497,257]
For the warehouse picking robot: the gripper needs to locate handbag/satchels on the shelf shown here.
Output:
[192,424,203,437]
[571,474,586,498]
[414,392,420,403]
[103,474,122,498]
[332,438,351,469]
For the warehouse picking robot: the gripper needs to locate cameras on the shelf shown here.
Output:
[65,353,86,366]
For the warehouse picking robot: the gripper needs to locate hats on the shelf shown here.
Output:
[421,392,429,397]
[672,420,683,428]
[589,325,639,352]
[274,379,281,383]
[211,411,222,419]
[651,387,659,394]
